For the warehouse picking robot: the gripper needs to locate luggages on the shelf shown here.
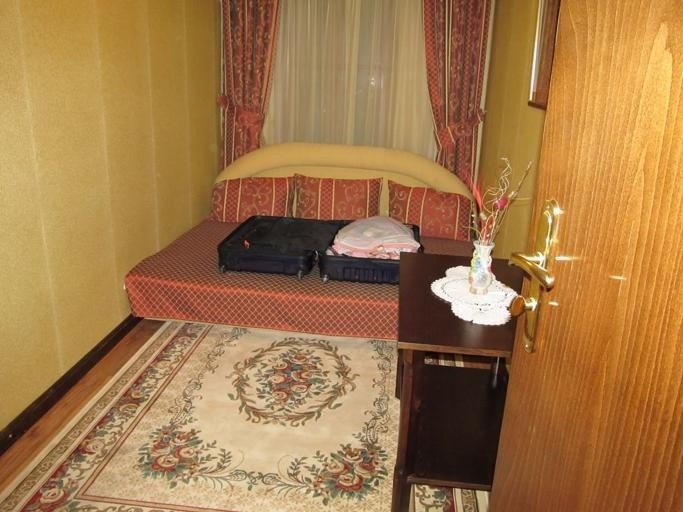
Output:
[216,215,426,286]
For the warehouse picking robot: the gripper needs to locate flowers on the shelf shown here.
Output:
[461,155,533,245]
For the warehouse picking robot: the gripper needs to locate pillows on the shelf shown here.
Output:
[292,175,388,221]
[212,173,297,222]
[386,178,473,244]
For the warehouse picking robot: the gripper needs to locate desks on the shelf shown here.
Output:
[392,251,534,511]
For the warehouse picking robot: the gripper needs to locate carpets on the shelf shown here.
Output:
[2,316,492,511]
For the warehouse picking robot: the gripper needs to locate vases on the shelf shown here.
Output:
[470,238,496,301]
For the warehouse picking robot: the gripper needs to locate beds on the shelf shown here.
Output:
[124,141,491,339]
